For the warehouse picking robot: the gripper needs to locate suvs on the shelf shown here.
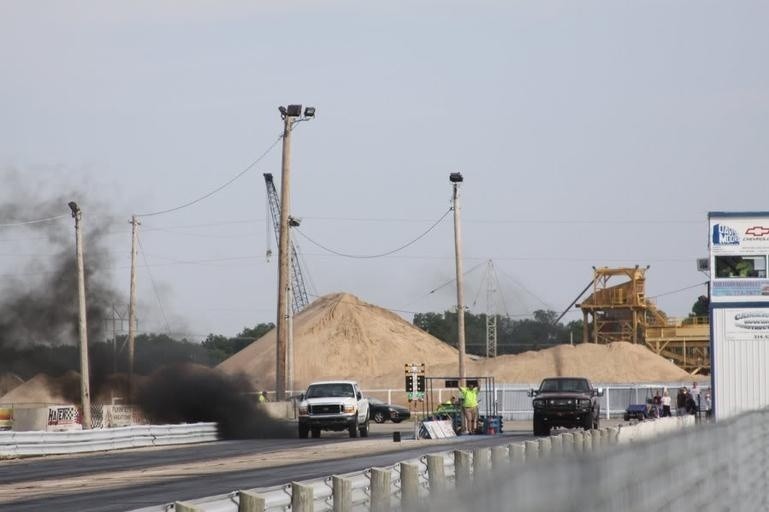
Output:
[524,375,606,440]
[295,380,373,437]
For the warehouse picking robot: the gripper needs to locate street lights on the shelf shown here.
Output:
[449,168,468,391]
[67,199,93,433]
[274,101,319,405]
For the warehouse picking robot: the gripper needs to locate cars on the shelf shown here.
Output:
[364,395,411,424]
[623,402,648,423]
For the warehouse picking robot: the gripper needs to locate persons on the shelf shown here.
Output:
[457,380,482,435]
[256,391,267,402]
[653,381,711,418]
[444,396,455,405]
